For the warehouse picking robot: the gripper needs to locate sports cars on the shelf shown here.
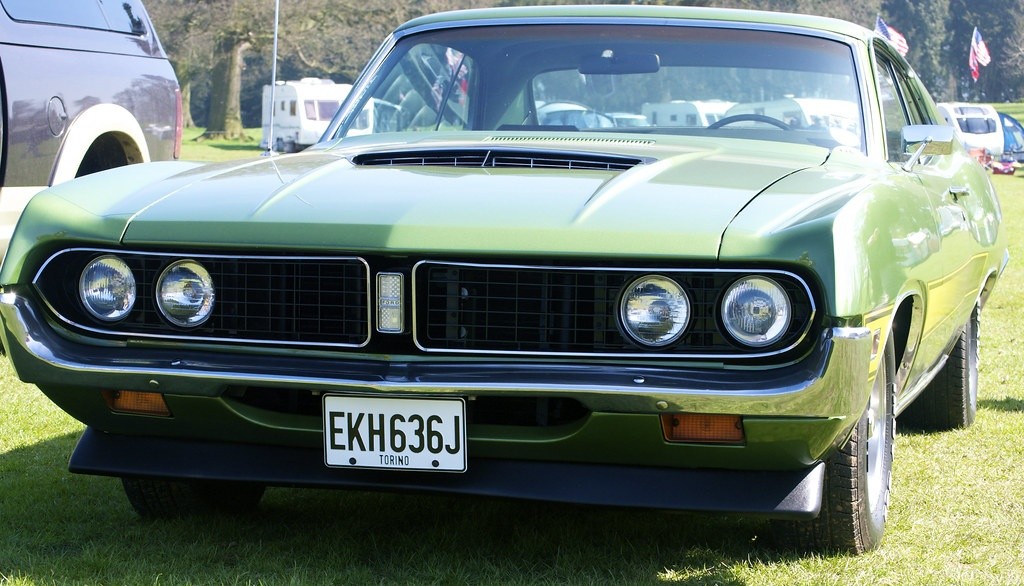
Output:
[0,8,1012,557]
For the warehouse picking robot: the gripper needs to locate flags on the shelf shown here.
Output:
[441,47,469,94]
[431,74,466,112]
[875,15,910,58]
[968,26,992,66]
[968,46,980,82]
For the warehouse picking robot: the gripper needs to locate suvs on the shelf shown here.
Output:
[0,2,184,274]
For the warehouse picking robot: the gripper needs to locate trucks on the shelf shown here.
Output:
[532,93,1006,155]
[261,76,375,154]
